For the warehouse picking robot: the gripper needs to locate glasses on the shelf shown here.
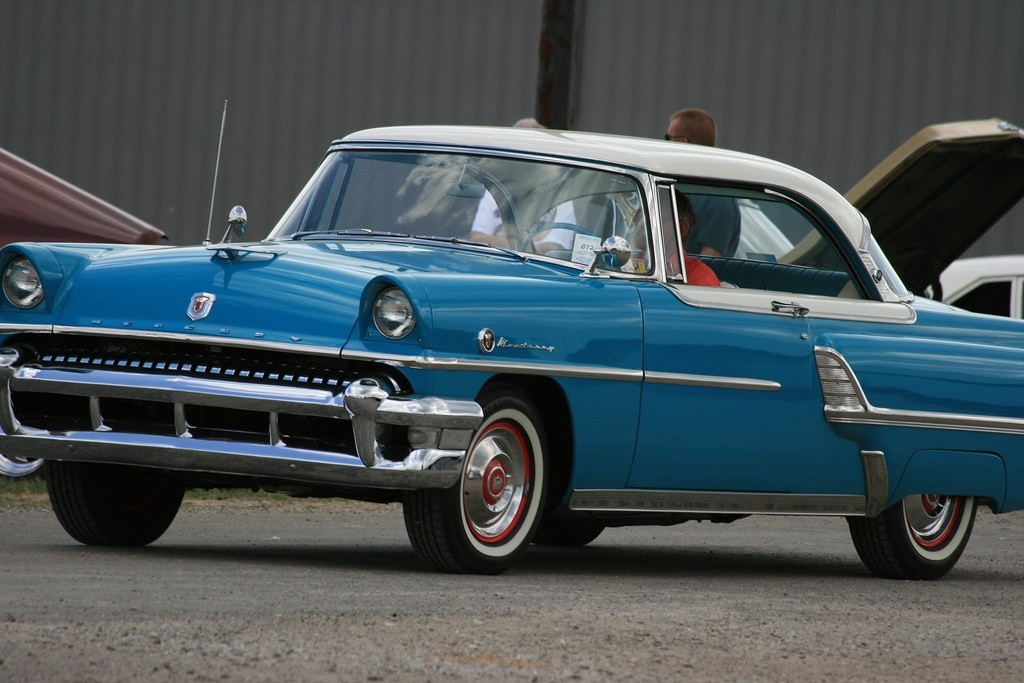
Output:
[665,134,689,140]
[633,206,674,224]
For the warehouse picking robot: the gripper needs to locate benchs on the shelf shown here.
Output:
[689,254,861,300]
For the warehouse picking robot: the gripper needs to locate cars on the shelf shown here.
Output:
[728,112,1024,321]
[0,126,1024,581]
[0,148,170,475]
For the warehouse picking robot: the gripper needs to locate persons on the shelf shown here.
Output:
[630,185,721,288]
[467,117,580,271]
[662,107,740,265]
